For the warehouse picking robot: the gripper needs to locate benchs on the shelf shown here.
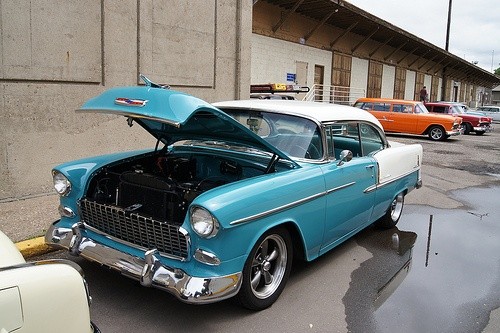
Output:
[268,134,381,158]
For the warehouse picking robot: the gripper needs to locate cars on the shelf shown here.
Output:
[426,102,493,136]
[462,105,487,117]
[352,98,463,142]
[476,106,500,121]
[248,83,348,136]
[0,229,102,333]
[44,73,423,313]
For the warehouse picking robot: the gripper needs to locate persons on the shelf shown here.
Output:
[420,86,426,102]
